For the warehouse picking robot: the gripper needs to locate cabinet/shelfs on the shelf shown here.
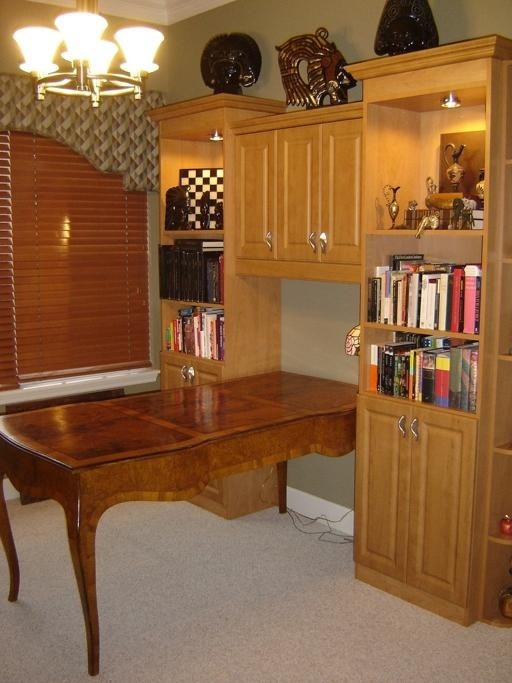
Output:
[362,85,479,417]
[159,354,228,522]
[474,58,510,632]
[358,397,471,622]
[233,115,359,266]
[150,94,223,363]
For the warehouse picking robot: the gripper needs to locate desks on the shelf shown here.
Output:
[0,369,360,676]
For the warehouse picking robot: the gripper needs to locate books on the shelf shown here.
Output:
[367,252,484,416]
[158,242,224,360]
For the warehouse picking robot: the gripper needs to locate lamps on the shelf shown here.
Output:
[11,1,166,110]
